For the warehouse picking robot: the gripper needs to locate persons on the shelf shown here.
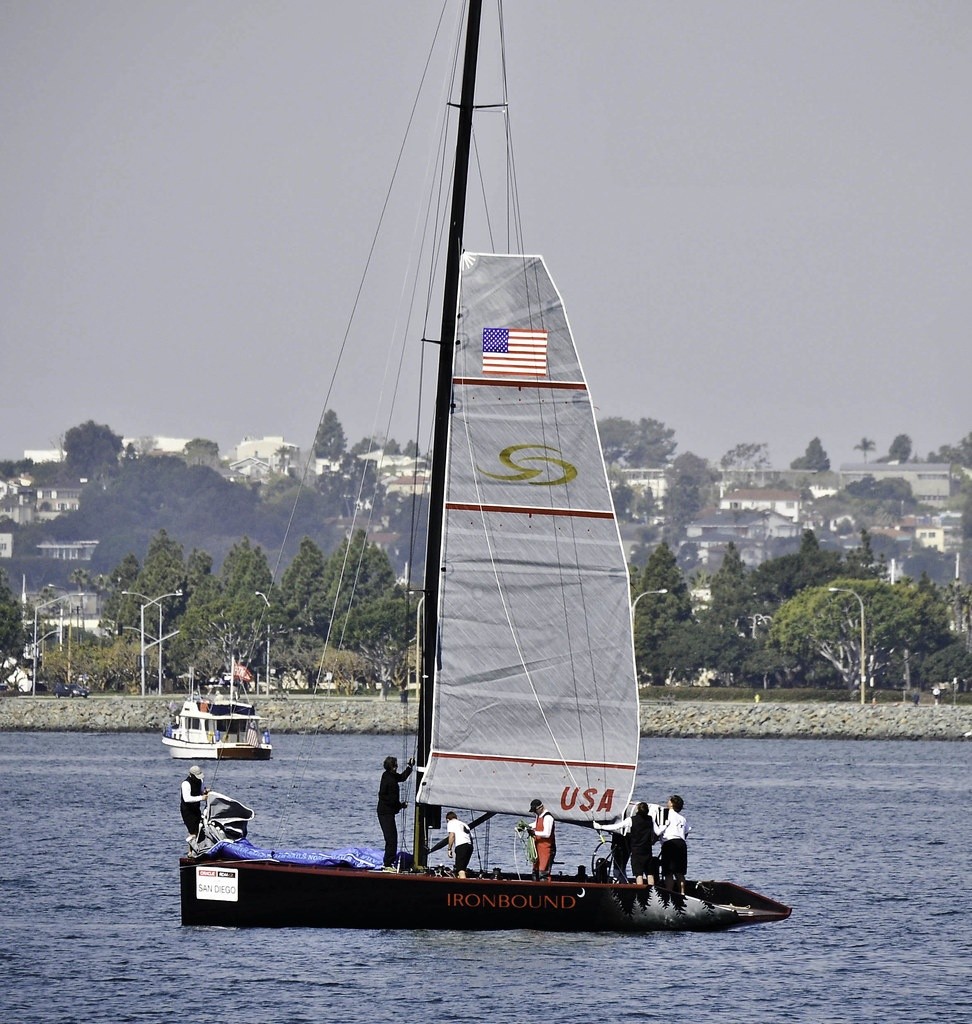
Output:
[181,766,212,835]
[914,693,920,705]
[658,795,691,895]
[593,802,670,885]
[754,692,761,705]
[376,755,415,872]
[446,811,473,879]
[517,800,557,882]
[933,687,941,704]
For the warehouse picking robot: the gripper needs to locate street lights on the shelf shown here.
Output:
[48,584,71,683]
[632,589,667,627]
[255,591,271,694]
[33,593,85,697]
[141,592,183,696]
[122,591,162,695]
[829,588,865,704]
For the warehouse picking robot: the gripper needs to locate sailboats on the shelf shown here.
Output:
[180,0,792,931]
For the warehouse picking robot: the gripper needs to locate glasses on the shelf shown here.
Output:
[394,764,399,768]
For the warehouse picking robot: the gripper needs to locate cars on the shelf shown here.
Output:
[52,684,90,698]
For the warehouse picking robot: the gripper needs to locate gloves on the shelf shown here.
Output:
[448,850,453,858]
[408,758,415,767]
[402,801,408,808]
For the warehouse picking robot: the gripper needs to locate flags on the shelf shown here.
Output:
[234,663,254,680]
[245,722,262,748]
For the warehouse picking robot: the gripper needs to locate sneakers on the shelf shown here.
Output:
[381,864,397,873]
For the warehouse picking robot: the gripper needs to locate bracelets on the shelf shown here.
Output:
[448,848,451,850]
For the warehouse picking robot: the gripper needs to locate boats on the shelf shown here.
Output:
[161,654,272,760]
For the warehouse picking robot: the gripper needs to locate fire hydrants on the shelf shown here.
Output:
[754,694,759,702]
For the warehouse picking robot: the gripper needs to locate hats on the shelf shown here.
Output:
[528,799,543,812]
[189,765,205,779]
